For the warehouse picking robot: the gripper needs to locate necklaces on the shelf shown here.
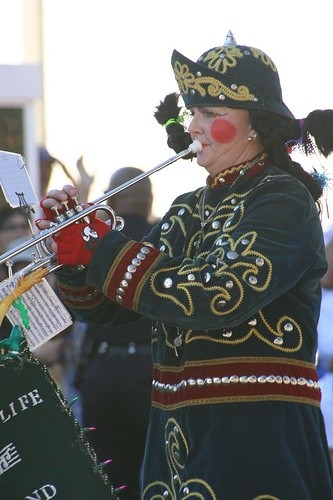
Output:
[192,156,263,259]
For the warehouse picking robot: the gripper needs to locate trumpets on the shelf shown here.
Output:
[0,139,202,285]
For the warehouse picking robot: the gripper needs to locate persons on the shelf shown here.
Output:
[33,31,333,500]
[56,166,154,498]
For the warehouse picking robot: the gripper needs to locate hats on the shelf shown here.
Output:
[171,29,295,119]
